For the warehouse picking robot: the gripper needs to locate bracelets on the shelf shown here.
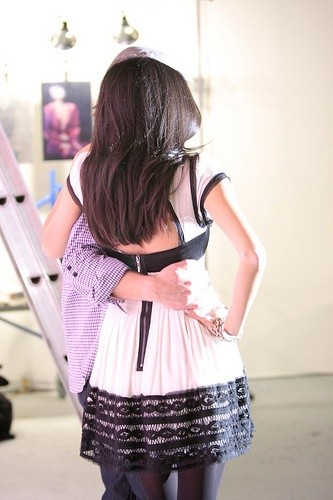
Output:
[211,317,224,339]
[221,324,243,342]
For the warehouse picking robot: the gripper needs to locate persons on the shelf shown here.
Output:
[38,56,267,500]
[60,46,213,500]
[44,85,89,158]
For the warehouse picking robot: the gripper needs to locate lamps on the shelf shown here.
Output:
[49,21,77,50]
[112,15,139,45]
